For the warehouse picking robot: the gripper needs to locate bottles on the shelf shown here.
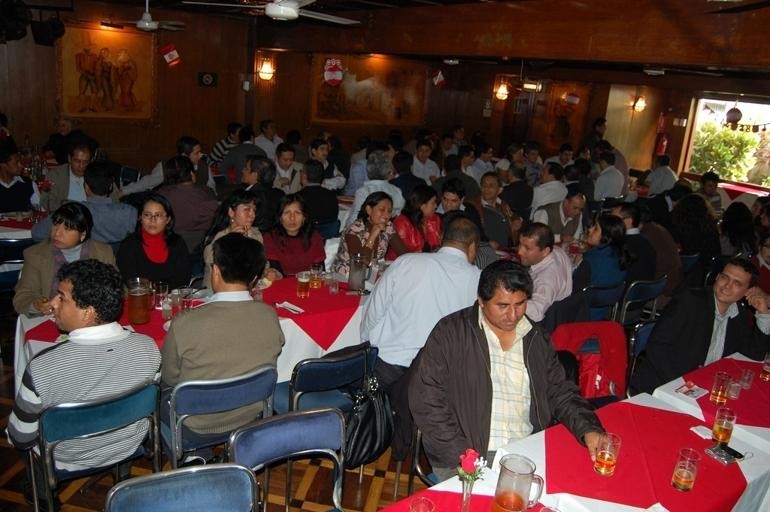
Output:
[16,134,48,182]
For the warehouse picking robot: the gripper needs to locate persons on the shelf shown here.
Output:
[408,259,615,482]
[1,114,346,317]
[331,122,629,323]
[629,256,769,398]
[159,231,287,468]
[4,259,161,477]
[610,152,769,310]
[360,216,484,461]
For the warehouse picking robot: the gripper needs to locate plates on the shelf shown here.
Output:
[162,320,171,331]
[495,250,509,259]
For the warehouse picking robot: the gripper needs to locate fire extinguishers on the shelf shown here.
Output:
[656,134,667,156]
[656,112,665,134]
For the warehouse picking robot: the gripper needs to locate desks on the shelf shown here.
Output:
[374,392,770,512]
[654,351,770,454]
[497,240,592,272]
[0,210,51,238]
[13,260,397,455]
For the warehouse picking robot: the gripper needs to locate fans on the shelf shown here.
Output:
[106,0,185,32]
[184,0,362,25]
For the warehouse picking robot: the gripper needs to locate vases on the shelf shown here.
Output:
[459,478,474,511]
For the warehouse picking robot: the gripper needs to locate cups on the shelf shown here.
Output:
[294,263,341,299]
[539,505,562,512]
[709,362,770,406]
[711,407,737,446]
[154,280,213,320]
[669,449,701,493]
[0,207,41,224]
[409,496,436,512]
[592,431,622,477]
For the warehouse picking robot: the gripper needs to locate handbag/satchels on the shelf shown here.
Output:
[344,381,395,471]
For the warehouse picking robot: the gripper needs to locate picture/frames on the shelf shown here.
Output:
[53,17,160,126]
[308,53,431,133]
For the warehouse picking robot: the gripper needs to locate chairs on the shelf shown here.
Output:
[603,195,624,214]
[273,347,379,485]
[591,198,605,225]
[120,166,141,187]
[1,239,39,316]
[160,364,278,469]
[407,427,440,495]
[103,464,259,512]
[314,219,341,239]
[541,249,743,410]
[27,380,160,512]
[229,407,347,512]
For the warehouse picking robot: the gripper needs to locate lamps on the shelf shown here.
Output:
[258,57,274,80]
[0,1,68,47]
[517,60,544,94]
[633,96,646,113]
[494,76,513,101]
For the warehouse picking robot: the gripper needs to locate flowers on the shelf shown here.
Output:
[455,448,487,488]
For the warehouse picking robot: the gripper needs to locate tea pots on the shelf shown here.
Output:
[493,454,544,512]
[348,254,371,292]
[123,277,156,324]
